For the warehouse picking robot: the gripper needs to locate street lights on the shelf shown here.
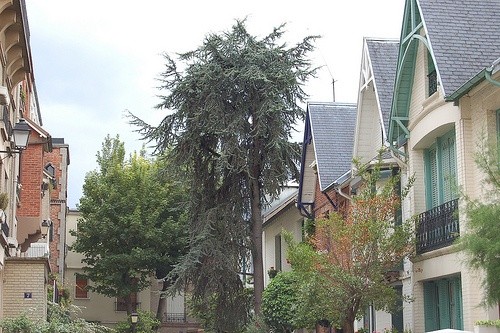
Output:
[129,313,139,333]
